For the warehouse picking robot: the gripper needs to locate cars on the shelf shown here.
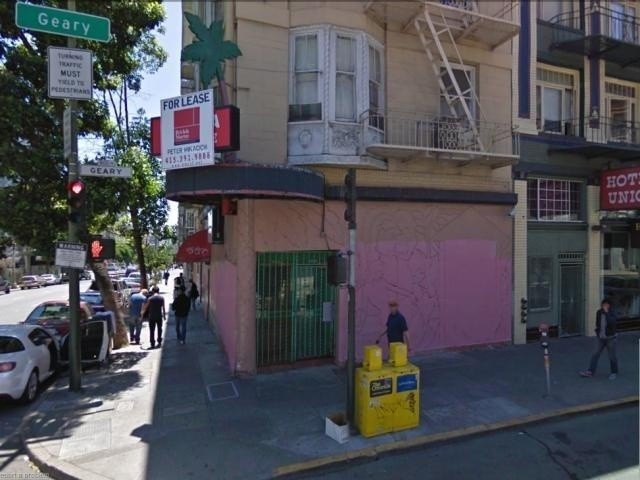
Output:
[17,261,153,341]
[0,276,11,294]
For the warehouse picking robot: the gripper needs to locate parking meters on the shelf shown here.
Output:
[537,322,555,394]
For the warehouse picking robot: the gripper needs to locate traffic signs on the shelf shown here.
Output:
[46,44,94,101]
[77,162,136,179]
[14,0,112,45]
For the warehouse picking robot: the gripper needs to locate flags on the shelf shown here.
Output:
[174,229,210,262]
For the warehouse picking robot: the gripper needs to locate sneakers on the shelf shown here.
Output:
[610,373,617,379]
[579,370,592,377]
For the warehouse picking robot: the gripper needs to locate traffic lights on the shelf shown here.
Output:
[343,167,358,230]
[87,237,116,261]
[66,177,88,212]
[325,254,349,285]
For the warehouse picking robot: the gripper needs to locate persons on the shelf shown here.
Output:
[375,298,411,359]
[576,298,618,380]
[164,270,170,285]
[143,287,166,348]
[127,288,149,344]
[170,273,199,344]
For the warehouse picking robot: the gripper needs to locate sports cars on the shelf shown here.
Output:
[0,318,111,407]
